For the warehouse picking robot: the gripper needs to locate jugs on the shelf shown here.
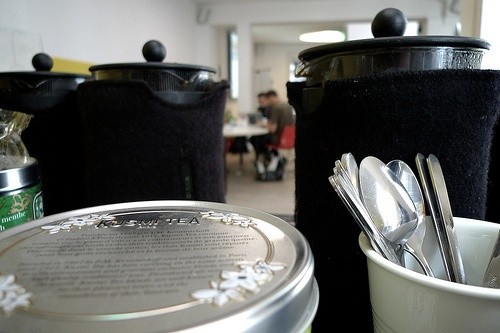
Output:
[0,53,92,216]
[75,39,226,203]
[284,8,493,280]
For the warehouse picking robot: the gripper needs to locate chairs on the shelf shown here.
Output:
[268,124,295,167]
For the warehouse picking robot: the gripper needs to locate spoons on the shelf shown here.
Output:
[359,155,435,281]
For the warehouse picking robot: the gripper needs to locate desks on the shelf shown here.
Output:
[223,122,270,169]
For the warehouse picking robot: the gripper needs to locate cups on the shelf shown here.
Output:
[359,216,500,333]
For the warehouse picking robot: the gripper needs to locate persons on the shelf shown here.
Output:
[251,92,277,162]
[250,90,295,173]
